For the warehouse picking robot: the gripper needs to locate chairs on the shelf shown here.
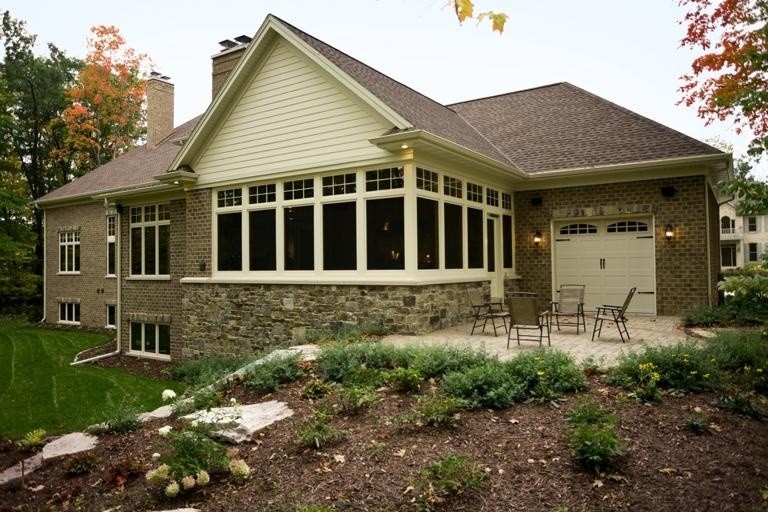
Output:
[467,290,509,337]
[592,287,636,342]
[506,292,552,348]
[550,284,586,335]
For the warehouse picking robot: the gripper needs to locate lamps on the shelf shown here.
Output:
[664,225,674,240]
[533,232,542,247]
